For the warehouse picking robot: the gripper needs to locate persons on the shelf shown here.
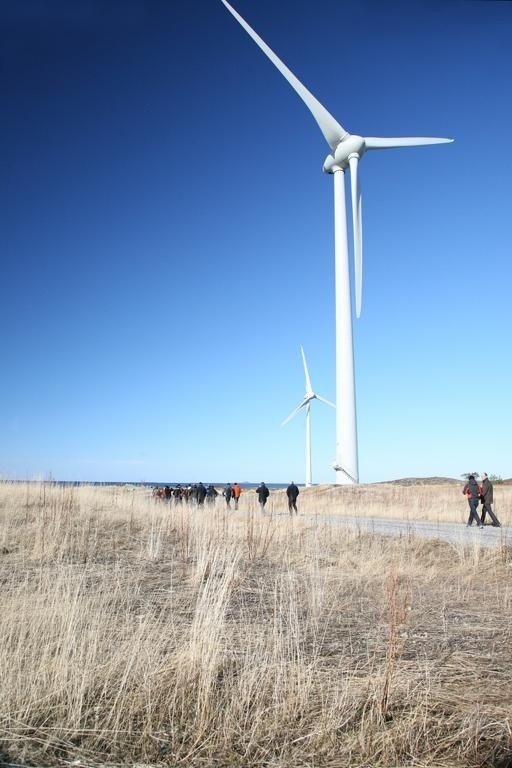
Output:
[476,472,500,528]
[255,481,270,516]
[286,481,298,516]
[461,475,484,529]
[149,480,242,510]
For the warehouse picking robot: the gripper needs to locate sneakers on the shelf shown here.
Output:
[465,522,501,529]
